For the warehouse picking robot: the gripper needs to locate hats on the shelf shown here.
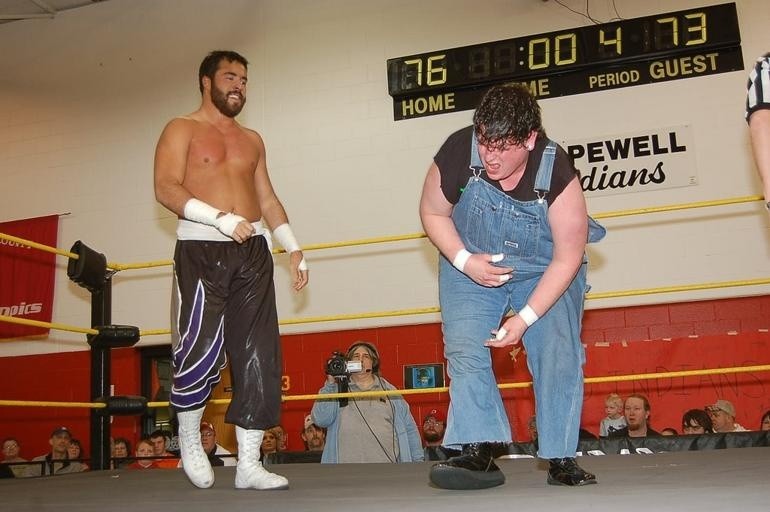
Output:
[704,400,735,418]
[422,408,445,427]
[51,426,71,439]
[344,341,380,361]
[304,414,314,430]
[199,420,214,432]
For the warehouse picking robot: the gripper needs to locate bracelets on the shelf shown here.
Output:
[452,250,471,275]
[520,303,539,325]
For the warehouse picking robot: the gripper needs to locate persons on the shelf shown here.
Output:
[745,52,769,214]
[421,391,770,445]
[313,341,426,463]
[0,416,325,478]
[420,85,605,489]
[153,51,309,487]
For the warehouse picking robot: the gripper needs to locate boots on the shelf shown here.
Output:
[547,457,597,486]
[429,442,505,489]
[235,424,289,490]
[176,404,215,489]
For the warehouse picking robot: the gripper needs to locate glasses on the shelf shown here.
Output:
[682,425,702,431]
